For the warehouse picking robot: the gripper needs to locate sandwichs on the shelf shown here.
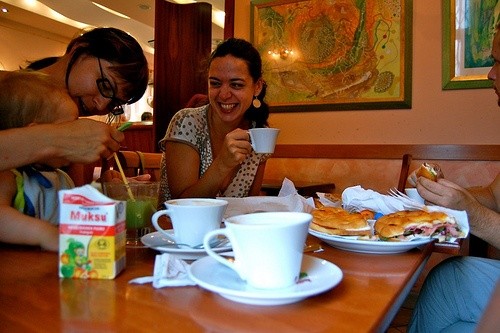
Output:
[374,210,460,242]
[416,162,438,183]
[310,206,372,237]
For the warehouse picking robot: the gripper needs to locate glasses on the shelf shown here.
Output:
[96,57,124,115]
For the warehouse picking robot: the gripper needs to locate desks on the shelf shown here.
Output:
[260,178,335,199]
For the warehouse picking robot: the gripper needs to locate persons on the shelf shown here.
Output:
[406,20,500,333]
[141,112,153,122]
[157,38,271,210]
[0,27,149,252]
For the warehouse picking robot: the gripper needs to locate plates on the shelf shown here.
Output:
[140,229,232,259]
[186,251,343,306]
[308,226,435,254]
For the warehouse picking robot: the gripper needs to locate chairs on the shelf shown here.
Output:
[396,150,412,195]
[102,151,164,183]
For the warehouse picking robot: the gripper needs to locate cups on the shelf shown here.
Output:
[151,198,229,251]
[103,181,160,248]
[246,127,280,154]
[405,188,424,204]
[203,212,313,289]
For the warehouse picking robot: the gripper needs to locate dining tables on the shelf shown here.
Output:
[0,195,435,333]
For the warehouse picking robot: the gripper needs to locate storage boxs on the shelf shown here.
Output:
[58,184,133,282]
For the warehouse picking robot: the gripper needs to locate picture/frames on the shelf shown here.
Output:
[249,0,413,114]
[441,0,500,91]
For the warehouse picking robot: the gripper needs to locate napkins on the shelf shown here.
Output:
[151,253,198,289]
[342,183,405,216]
